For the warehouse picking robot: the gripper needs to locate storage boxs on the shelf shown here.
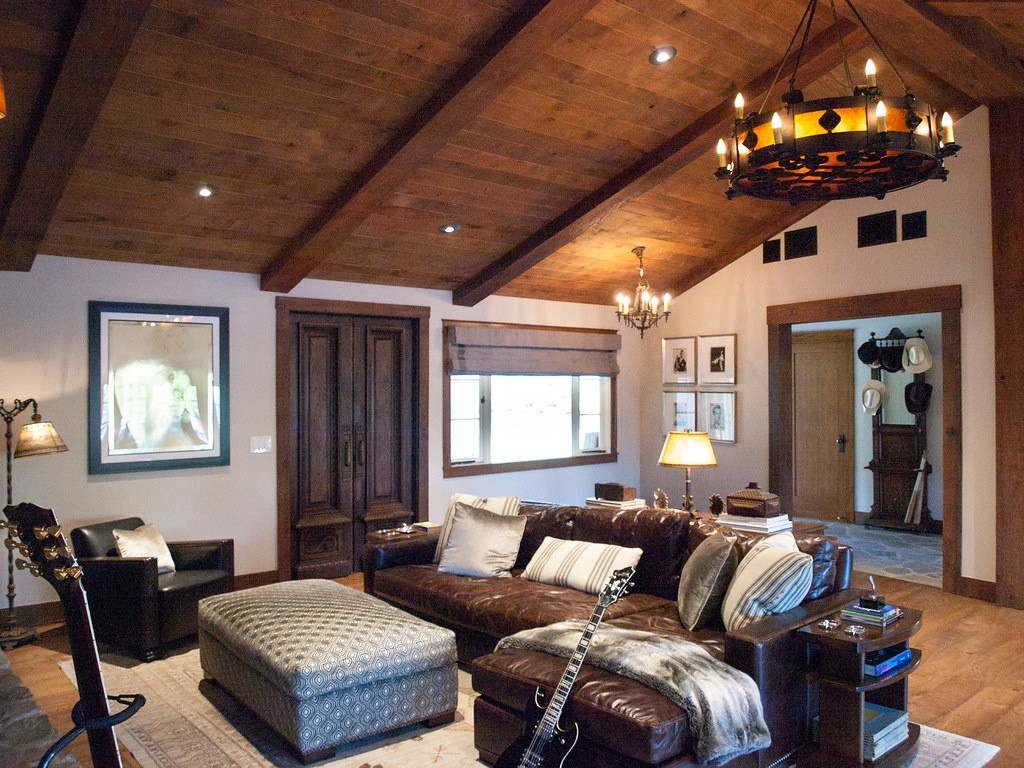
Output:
[727,482,793,517]
[595,483,636,502]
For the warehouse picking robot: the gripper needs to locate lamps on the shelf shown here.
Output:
[713,0,963,206]
[657,429,718,520]
[0,399,69,651]
[614,246,672,339]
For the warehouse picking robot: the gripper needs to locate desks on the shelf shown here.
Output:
[696,512,828,537]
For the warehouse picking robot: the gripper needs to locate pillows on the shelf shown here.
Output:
[111,523,175,574]
[677,532,738,632]
[521,536,643,596]
[432,493,527,578]
[721,531,814,632]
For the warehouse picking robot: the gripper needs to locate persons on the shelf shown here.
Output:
[712,350,725,371]
[674,349,688,372]
[712,403,722,430]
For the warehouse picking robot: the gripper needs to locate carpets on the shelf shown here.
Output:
[58,636,1001,768]
[0,649,82,768]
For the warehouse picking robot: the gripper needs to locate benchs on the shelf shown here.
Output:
[197,579,458,767]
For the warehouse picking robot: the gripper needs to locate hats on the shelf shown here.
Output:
[861,379,886,416]
[901,338,932,374]
[904,382,933,415]
[857,327,907,373]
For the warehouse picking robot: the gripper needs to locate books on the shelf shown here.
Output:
[864,645,912,677]
[864,701,910,760]
[840,598,904,627]
[585,496,646,510]
[715,511,793,535]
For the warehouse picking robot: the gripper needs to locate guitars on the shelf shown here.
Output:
[2,499,123,768]
[492,564,639,768]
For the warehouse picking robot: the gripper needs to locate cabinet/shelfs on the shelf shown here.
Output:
[864,328,937,535]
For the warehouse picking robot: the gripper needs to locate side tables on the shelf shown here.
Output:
[797,600,922,768]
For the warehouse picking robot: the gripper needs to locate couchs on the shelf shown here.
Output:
[365,501,716,675]
[471,533,877,768]
[70,517,235,663]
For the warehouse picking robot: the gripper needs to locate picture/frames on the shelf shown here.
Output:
[88,300,230,475]
[660,333,737,444]
